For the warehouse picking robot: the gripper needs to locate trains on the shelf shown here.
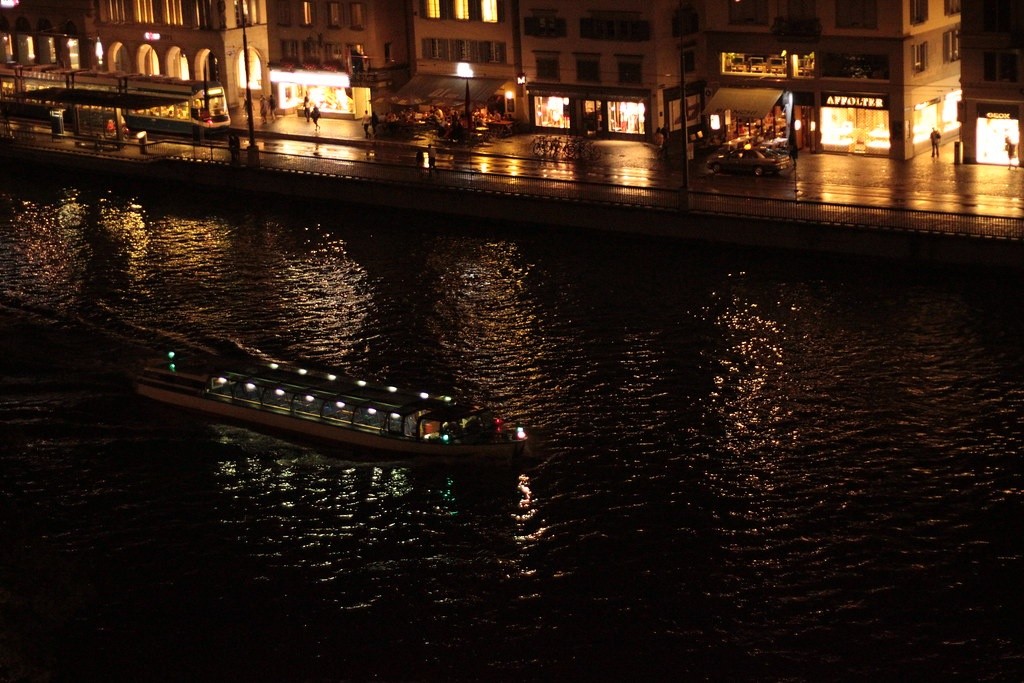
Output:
[1,61,232,140]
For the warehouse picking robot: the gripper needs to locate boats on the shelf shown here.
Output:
[123,351,565,473]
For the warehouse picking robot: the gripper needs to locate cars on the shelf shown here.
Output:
[707,148,791,176]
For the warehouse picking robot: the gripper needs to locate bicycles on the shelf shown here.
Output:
[530,135,600,161]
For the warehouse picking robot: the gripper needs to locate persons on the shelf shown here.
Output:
[427,144,439,176]
[268,94,276,123]
[260,98,268,124]
[930,126,941,157]
[242,100,256,121]
[311,106,321,130]
[360,106,514,146]
[303,96,311,122]
[105,99,221,139]
[655,127,664,161]
[661,122,670,158]
[416,147,425,177]
[1006,138,1018,171]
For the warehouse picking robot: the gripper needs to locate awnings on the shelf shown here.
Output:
[385,73,508,112]
[4,86,188,151]
[701,87,784,119]
